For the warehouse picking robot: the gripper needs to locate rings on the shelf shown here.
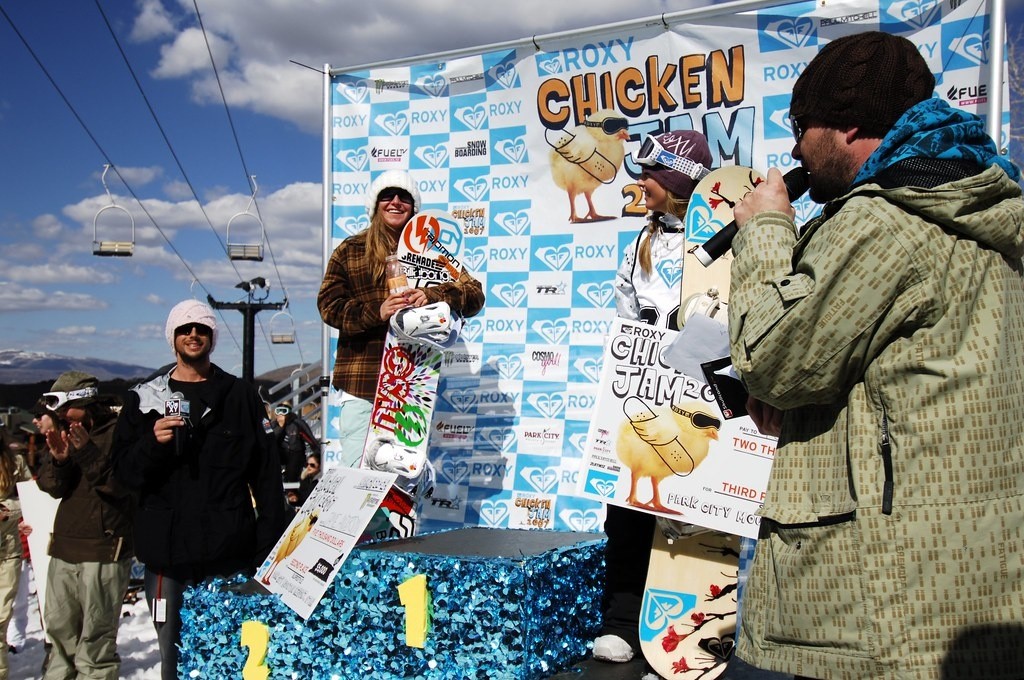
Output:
[76,438,80,442]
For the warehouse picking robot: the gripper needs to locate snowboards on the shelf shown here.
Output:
[351,209,465,548]
[638,164,783,680]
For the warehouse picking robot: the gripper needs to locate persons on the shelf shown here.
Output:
[270,401,321,506]
[110,300,287,680]
[727,31,1024,680]
[317,169,485,468]
[0,370,136,680]
[592,130,713,663]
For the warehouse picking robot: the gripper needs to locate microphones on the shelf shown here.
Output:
[692,167,811,268]
[164,391,190,457]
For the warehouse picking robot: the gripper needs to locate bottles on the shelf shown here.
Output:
[385,255,410,300]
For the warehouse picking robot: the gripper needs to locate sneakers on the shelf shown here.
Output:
[593,634,634,663]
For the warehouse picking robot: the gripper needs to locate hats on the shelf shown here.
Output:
[644,127,714,199]
[367,170,422,220]
[165,300,218,356]
[49,371,100,408]
[789,29,936,135]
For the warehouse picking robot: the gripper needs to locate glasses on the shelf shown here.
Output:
[42,386,98,410]
[790,114,810,141]
[304,463,316,469]
[636,134,711,183]
[275,407,290,416]
[379,191,413,205]
[177,325,208,336]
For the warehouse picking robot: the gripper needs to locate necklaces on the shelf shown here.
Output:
[660,223,684,247]
